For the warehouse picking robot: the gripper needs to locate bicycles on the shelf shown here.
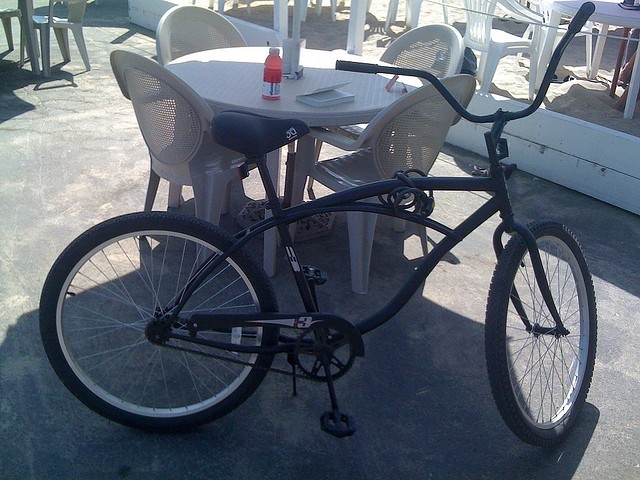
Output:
[37,2,595,447]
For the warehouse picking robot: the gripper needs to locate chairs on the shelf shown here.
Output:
[155,4,249,66]
[289,74,477,295]
[33,0,91,78]
[461,1,531,94]
[378,24,464,104]
[109,49,263,270]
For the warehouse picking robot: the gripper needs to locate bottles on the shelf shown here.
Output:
[262,48,283,100]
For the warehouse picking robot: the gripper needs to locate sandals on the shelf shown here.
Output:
[552,74,574,83]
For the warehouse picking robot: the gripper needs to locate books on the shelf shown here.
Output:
[295,80,357,108]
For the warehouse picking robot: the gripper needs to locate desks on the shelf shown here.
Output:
[534,0,639,120]
[161,47,415,277]
